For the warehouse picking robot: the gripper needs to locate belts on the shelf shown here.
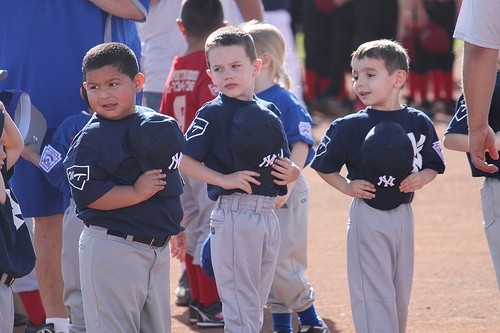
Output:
[84,223,171,248]
[0,272,15,287]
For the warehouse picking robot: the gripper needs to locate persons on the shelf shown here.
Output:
[0,0,151,333]
[453,0,500,173]
[178,26,300,333]
[310,40,446,333]
[159,0,228,327]
[261,0,463,128]
[443,46,500,291]
[239,20,329,333]
[63,42,189,333]
[0,69,64,333]
[136,0,263,306]
[20,82,95,333]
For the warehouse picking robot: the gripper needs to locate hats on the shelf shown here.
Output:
[0,70,7,79]
[129,112,184,197]
[0,90,48,154]
[229,104,283,192]
[358,121,414,210]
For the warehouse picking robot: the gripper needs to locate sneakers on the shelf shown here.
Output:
[35,322,66,333]
[176,287,191,307]
[197,302,225,326]
[297,318,328,333]
[189,299,199,322]
